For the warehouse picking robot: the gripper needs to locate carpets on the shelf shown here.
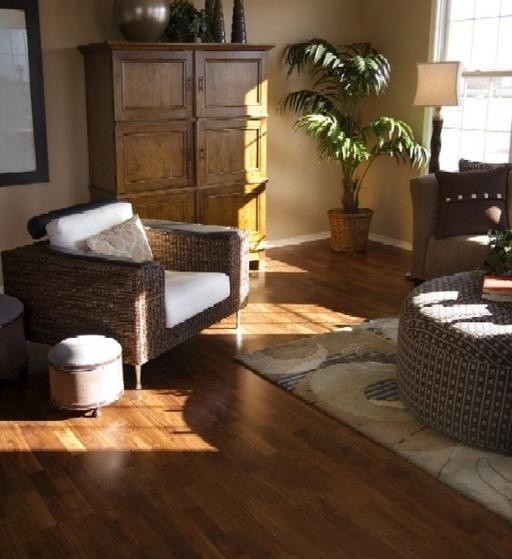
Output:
[234,315,511,523]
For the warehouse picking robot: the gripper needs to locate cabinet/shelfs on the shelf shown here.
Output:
[77,40,276,270]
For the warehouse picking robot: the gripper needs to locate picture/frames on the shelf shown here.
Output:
[0,0,49,188]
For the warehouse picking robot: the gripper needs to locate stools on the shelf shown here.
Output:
[0,294,27,381]
[398,271,511,455]
[49,335,124,417]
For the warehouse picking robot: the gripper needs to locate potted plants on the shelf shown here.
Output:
[190,9,206,43]
[277,38,431,254]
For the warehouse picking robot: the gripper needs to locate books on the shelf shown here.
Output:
[480,274,512,302]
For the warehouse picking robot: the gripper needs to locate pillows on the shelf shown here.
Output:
[28,198,154,264]
[461,158,511,175]
[436,169,508,238]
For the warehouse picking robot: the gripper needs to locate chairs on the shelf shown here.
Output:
[0,219,249,389]
[406,175,512,280]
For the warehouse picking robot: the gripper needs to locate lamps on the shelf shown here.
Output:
[412,62,459,174]
